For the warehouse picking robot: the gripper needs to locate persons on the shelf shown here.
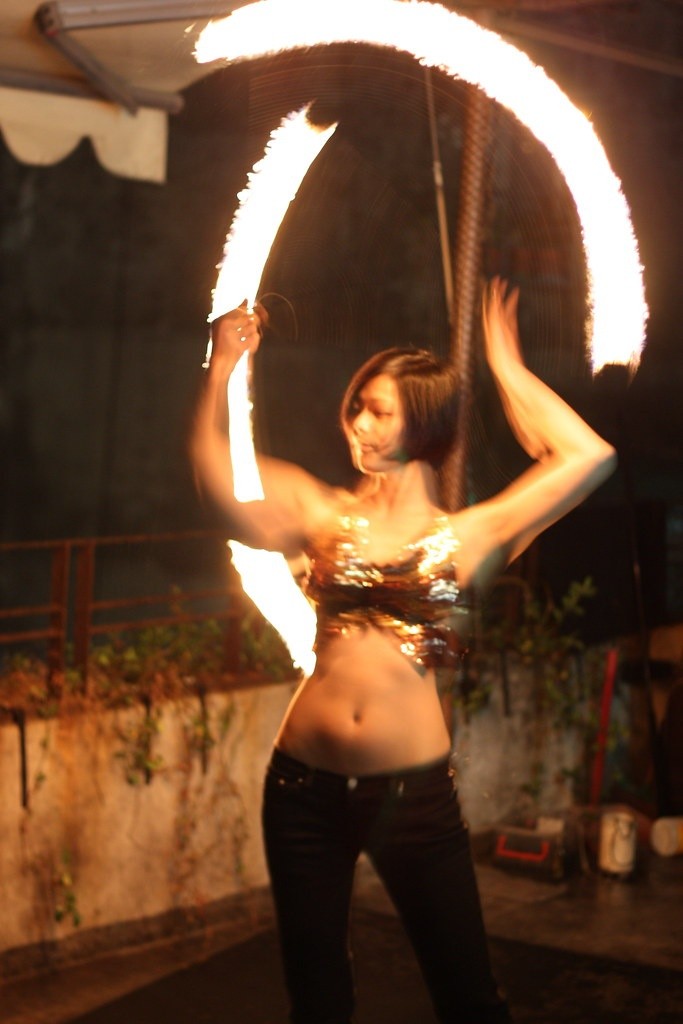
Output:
[188,275,618,1024]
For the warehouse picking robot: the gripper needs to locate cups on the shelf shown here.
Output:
[597,810,637,873]
